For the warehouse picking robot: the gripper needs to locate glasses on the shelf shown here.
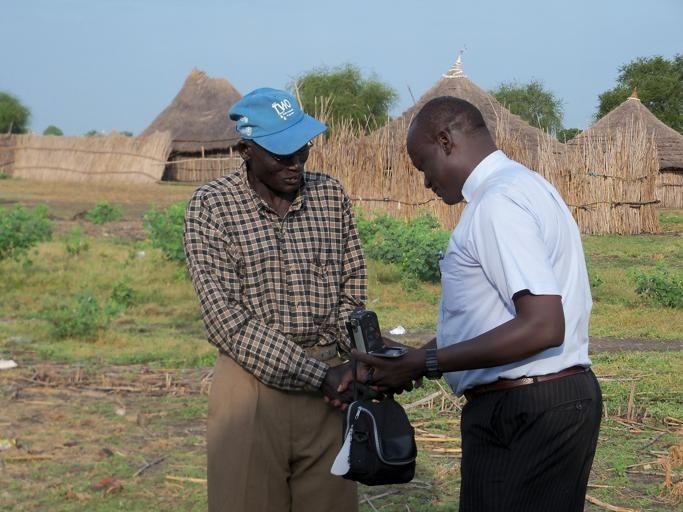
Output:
[260,141,314,163]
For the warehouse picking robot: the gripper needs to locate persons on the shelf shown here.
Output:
[182,87,424,510]
[322,94,601,511]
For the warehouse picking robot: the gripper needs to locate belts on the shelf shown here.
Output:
[463,364,589,402]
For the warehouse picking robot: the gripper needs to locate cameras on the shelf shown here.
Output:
[345,307,408,358]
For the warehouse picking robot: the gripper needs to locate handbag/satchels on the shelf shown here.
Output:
[334,392,417,486]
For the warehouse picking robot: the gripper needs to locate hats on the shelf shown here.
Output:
[228,88,328,156]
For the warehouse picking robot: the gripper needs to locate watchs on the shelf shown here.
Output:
[425,347,443,380]
